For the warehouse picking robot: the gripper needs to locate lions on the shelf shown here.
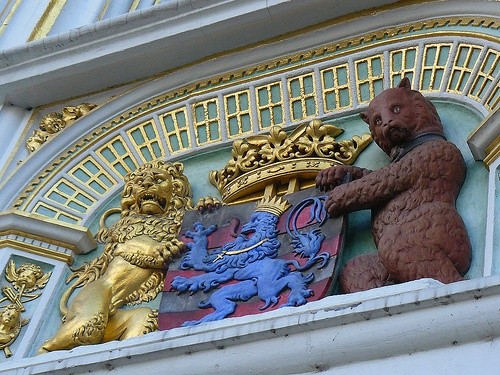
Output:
[36,150,222,355]
